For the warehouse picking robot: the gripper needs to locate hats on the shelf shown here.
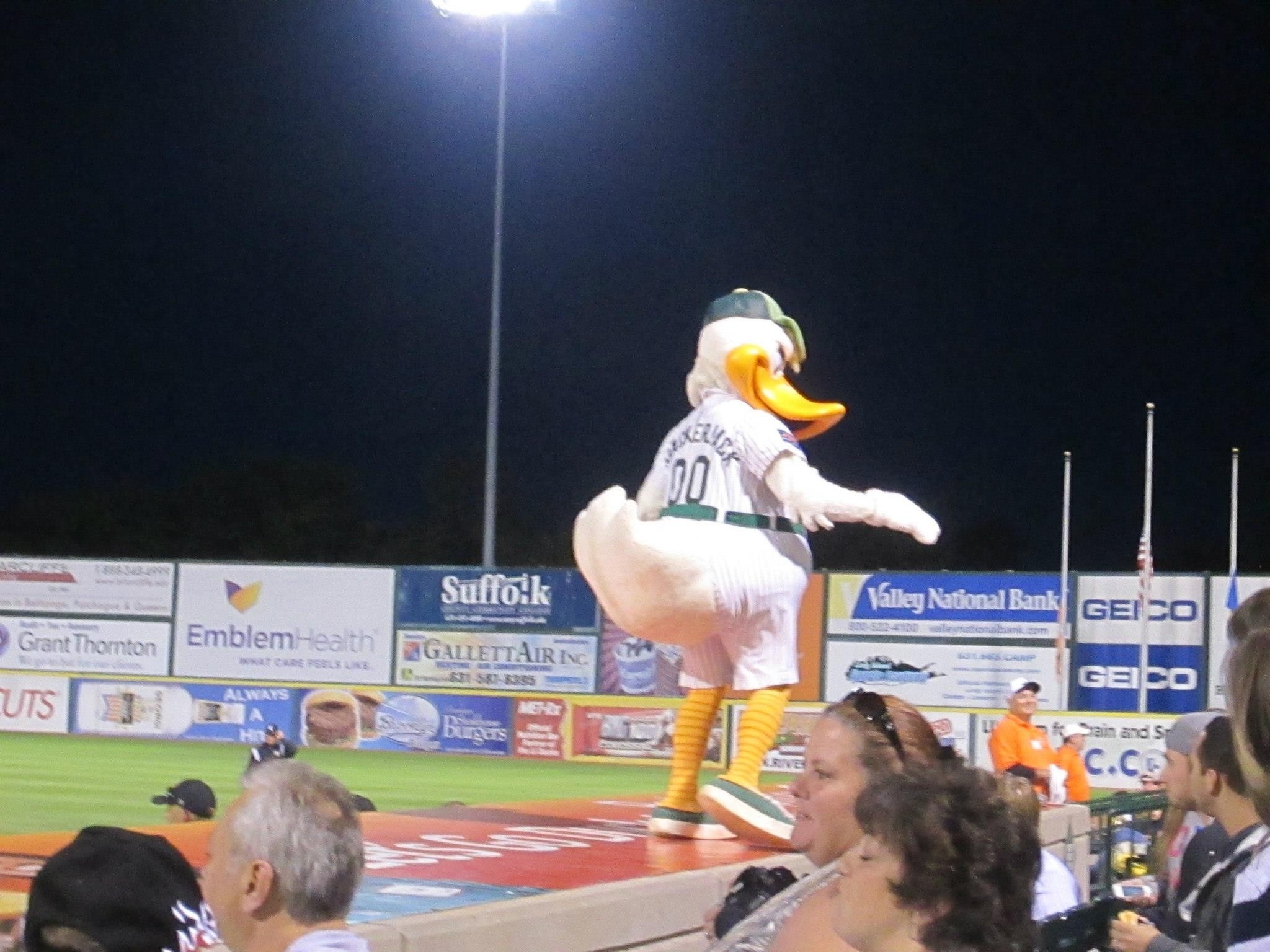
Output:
[1060,724,1091,739]
[265,722,280,737]
[1164,710,1222,756]
[150,778,216,819]
[23,824,203,952]
[1007,677,1041,698]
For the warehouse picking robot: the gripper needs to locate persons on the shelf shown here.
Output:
[827,758,1045,952]
[198,758,371,951]
[24,826,231,952]
[245,725,298,775]
[1108,585,1270,951]
[989,678,1059,807]
[705,688,965,952]
[153,780,216,823]
[1052,723,1092,804]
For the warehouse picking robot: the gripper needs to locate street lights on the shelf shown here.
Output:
[432,0,536,564]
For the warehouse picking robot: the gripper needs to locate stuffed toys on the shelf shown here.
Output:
[573,287,942,848]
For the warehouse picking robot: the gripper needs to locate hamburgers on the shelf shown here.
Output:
[304,689,387,750]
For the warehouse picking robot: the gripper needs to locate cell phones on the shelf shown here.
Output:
[1112,883,1152,902]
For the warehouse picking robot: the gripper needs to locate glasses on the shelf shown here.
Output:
[840,686,907,769]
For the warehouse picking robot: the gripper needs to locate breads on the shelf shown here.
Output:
[1117,910,1137,925]
